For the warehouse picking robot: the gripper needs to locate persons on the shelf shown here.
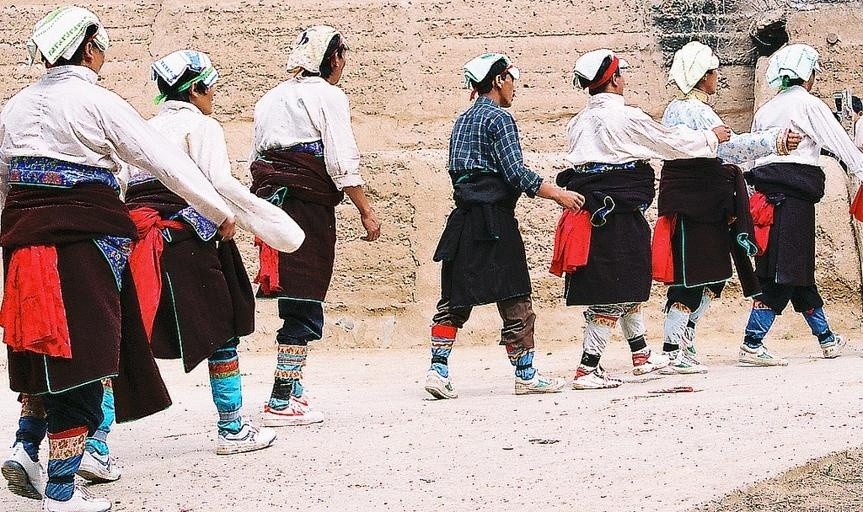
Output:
[558,49,731,392]
[75,49,276,482]
[424,53,565,399]
[243,25,382,427]
[0,6,237,512]
[844,95,863,148]
[734,44,863,367]
[650,40,802,375]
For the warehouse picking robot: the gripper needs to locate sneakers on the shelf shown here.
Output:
[263,401,323,426]
[739,343,788,367]
[631,347,709,376]
[216,423,277,455]
[2,442,117,512]
[573,365,622,389]
[425,370,457,399]
[821,335,847,357]
[515,370,565,394]
